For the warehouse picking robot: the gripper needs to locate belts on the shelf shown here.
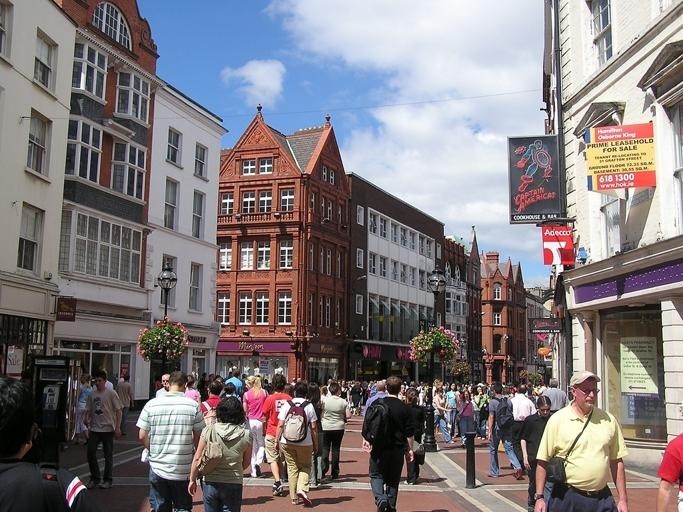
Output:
[571,485,607,498]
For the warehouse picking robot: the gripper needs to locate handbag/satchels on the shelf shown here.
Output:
[196,425,224,476]
[544,456,564,483]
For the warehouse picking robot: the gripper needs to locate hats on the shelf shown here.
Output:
[569,370,600,388]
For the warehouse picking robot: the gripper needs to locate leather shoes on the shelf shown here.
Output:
[377,499,388,512]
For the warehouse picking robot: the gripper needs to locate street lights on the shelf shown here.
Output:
[502,334,509,355]
[423,264,447,452]
[155,264,178,380]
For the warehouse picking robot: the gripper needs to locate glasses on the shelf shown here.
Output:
[576,387,599,397]
[30,423,38,440]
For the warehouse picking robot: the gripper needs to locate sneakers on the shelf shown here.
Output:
[291,498,298,505]
[272,485,283,496]
[296,489,313,507]
[85,480,97,489]
[98,480,113,489]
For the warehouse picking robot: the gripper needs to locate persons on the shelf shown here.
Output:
[0,378,92,511]
[518,396,554,510]
[656,433,683,511]
[133,372,206,511]
[75,366,132,444]
[84,370,122,488]
[532,371,630,511]
[151,366,568,507]
[187,397,251,512]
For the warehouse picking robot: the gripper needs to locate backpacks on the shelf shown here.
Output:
[202,401,217,426]
[361,397,394,444]
[283,400,312,443]
[491,397,513,429]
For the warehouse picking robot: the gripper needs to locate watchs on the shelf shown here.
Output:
[531,492,543,502]
